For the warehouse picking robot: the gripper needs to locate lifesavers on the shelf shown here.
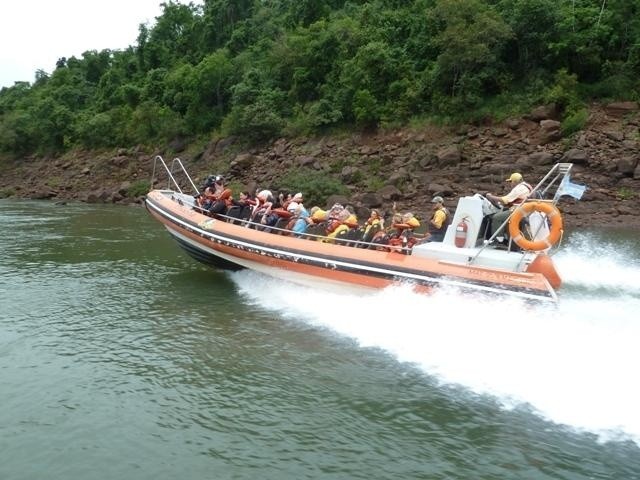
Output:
[509,202,563,250]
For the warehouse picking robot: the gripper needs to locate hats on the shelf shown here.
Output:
[430,196,445,203]
[214,175,224,182]
[505,173,524,182]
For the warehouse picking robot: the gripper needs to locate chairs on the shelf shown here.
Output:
[205,198,414,254]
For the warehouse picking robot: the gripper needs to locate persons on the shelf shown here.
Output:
[194,174,421,255]
[424,194,453,242]
[484,171,539,253]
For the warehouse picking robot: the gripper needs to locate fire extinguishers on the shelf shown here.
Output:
[455,216,470,248]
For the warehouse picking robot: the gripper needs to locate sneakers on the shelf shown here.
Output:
[490,240,505,247]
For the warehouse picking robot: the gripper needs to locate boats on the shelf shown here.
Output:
[143,156,574,304]
